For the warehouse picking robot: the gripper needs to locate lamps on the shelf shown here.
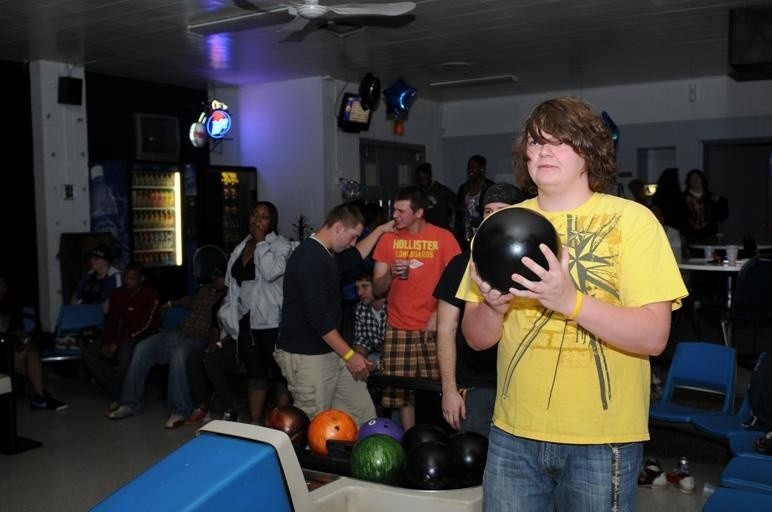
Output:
[189,6,295,41]
[430,75,518,89]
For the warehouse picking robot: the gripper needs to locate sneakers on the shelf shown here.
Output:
[31,391,69,411]
[221,409,240,422]
[105,400,138,418]
[665,471,694,494]
[165,407,210,428]
[638,467,667,487]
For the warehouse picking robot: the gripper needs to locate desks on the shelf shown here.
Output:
[679,254,751,349]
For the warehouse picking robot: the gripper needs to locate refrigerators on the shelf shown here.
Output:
[192,163,259,287]
[89,159,187,299]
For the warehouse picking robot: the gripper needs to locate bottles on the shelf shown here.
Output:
[379,199,391,221]
[133,172,177,266]
[183,164,196,195]
[224,181,239,226]
[90,164,119,253]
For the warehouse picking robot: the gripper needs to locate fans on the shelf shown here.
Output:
[245,1,416,36]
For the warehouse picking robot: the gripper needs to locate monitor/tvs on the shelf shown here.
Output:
[127,111,180,164]
[336,91,373,134]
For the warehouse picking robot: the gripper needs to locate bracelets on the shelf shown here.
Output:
[568,291,583,321]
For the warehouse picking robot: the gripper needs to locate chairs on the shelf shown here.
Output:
[44,261,230,393]
[649,339,772,512]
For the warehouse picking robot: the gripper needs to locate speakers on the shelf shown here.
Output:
[58,76,83,106]
[727,3,772,74]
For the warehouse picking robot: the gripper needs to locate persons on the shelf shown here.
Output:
[411,153,495,238]
[33,248,229,428]
[453,97,689,512]
[214,185,462,433]
[627,165,729,249]
[432,182,530,440]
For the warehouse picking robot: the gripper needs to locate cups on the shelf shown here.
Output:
[702,481,715,497]
[715,233,724,244]
[395,259,410,280]
[703,246,714,261]
[725,244,738,267]
